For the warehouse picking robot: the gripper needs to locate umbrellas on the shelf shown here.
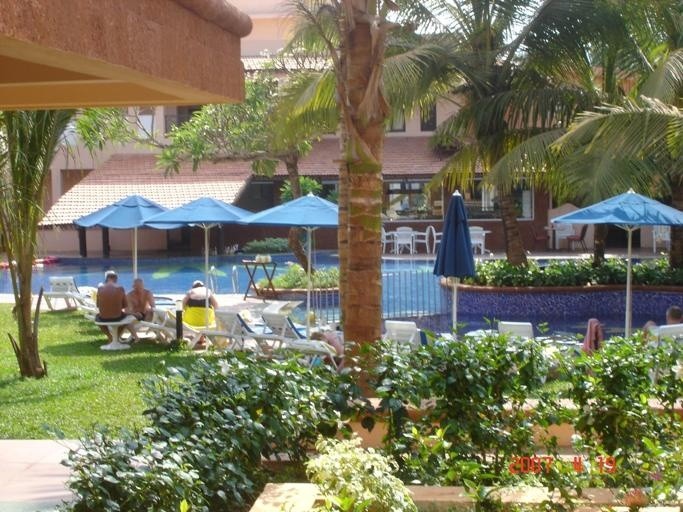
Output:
[138,191,257,352]
[239,189,338,336]
[426,188,478,341]
[70,192,171,278]
[548,186,683,340]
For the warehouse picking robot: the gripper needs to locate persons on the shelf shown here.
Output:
[125,276,158,345]
[638,304,683,337]
[309,330,344,366]
[92,269,129,343]
[178,278,220,351]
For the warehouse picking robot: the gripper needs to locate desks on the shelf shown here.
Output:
[242,260,276,302]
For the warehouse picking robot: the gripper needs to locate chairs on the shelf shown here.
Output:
[651,226,670,253]
[528,223,590,253]
[376,225,492,271]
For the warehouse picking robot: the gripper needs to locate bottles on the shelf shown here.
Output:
[254,254,270,263]
